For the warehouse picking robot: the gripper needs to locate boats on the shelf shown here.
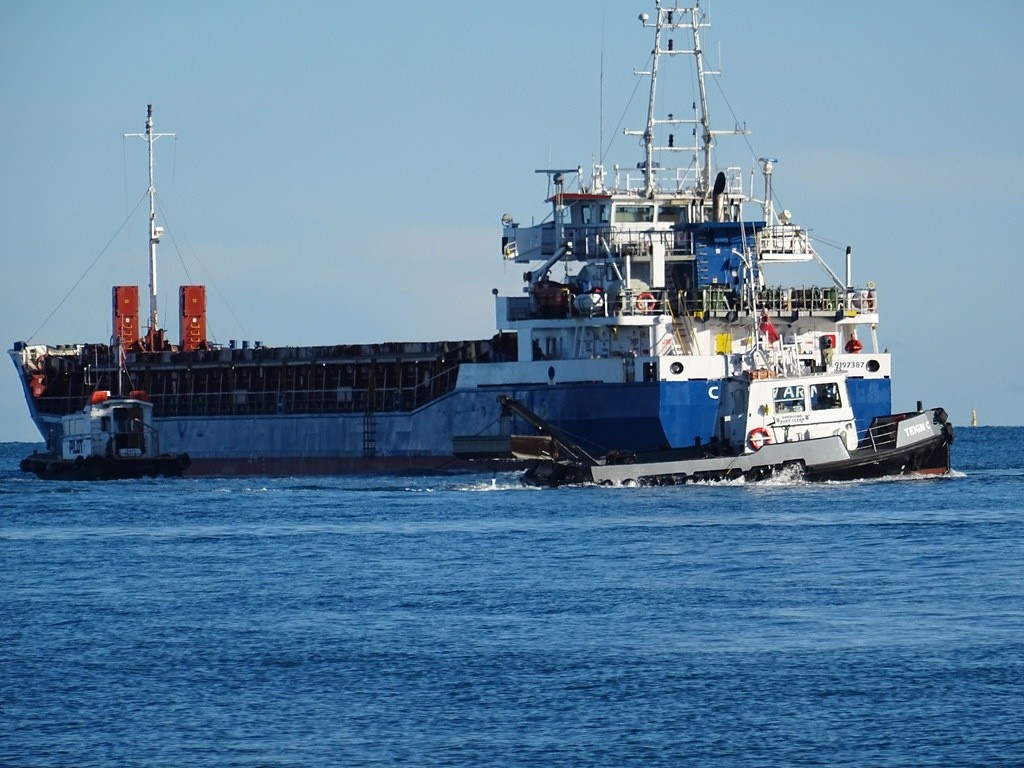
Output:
[497,394,952,489]
[19,313,192,482]
[7,0,890,487]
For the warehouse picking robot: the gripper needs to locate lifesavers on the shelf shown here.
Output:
[868,293,874,308]
[636,292,656,311]
[178,454,192,469]
[750,427,771,451]
